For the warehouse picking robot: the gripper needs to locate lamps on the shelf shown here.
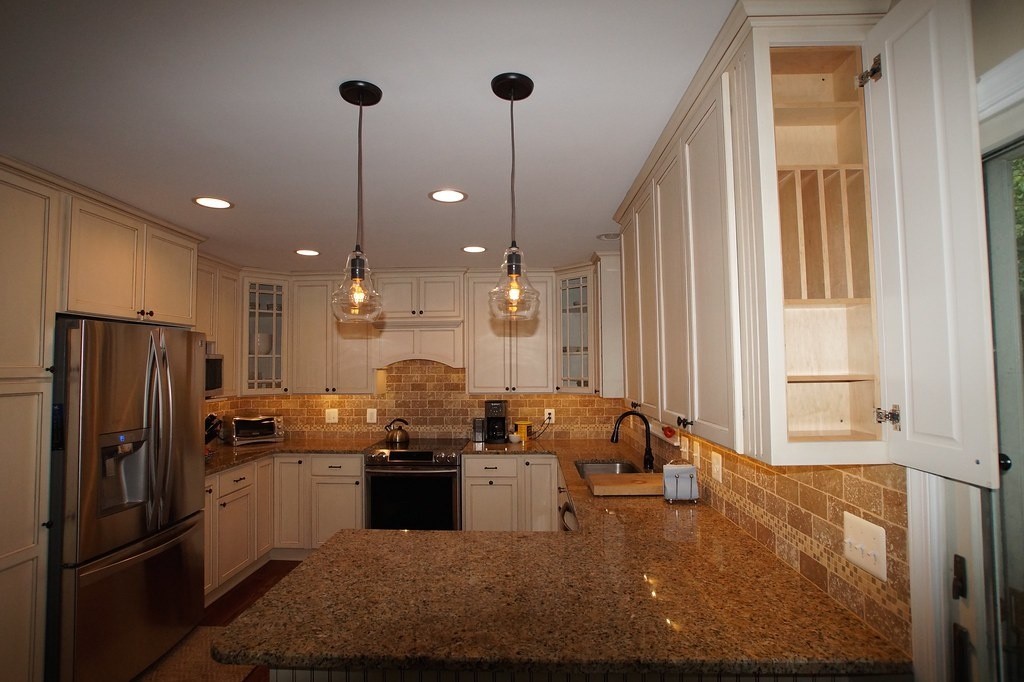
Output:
[331,80,383,323]
[489,73,539,321]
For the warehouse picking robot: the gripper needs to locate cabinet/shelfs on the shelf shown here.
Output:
[614,72,743,453]
[593,258,625,398]
[0,166,55,679]
[467,273,552,394]
[60,193,202,327]
[556,267,594,393]
[205,453,363,595]
[197,258,237,395]
[293,281,371,394]
[463,456,558,533]
[241,273,290,395]
[725,0,1002,489]
[374,271,464,323]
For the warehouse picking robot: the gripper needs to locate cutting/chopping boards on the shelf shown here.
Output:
[586,473,665,495]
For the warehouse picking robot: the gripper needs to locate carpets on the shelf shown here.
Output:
[131,626,257,682]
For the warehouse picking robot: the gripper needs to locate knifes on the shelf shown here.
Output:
[205,414,222,444]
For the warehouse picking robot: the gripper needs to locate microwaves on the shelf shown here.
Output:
[223,415,285,446]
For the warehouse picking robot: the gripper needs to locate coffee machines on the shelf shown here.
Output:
[484,400,507,443]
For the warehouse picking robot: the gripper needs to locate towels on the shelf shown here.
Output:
[662,464,699,501]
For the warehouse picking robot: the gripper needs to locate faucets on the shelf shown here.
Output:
[610,410,654,474]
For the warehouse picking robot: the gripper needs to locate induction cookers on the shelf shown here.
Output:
[364,438,471,465]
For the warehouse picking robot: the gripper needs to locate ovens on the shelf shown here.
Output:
[365,465,461,531]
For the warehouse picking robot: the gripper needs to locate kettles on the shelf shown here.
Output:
[385,418,409,442]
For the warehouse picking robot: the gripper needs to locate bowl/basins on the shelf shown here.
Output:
[509,435,521,443]
[249,302,260,309]
[267,304,281,311]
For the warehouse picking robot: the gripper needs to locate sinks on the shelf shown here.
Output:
[575,463,640,479]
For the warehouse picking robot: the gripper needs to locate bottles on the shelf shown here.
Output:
[473,419,484,442]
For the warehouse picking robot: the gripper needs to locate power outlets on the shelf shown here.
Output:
[545,408,555,423]
[711,451,722,483]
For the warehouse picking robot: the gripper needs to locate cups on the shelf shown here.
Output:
[257,334,272,354]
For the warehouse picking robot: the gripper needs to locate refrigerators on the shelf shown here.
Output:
[60,317,206,682]
